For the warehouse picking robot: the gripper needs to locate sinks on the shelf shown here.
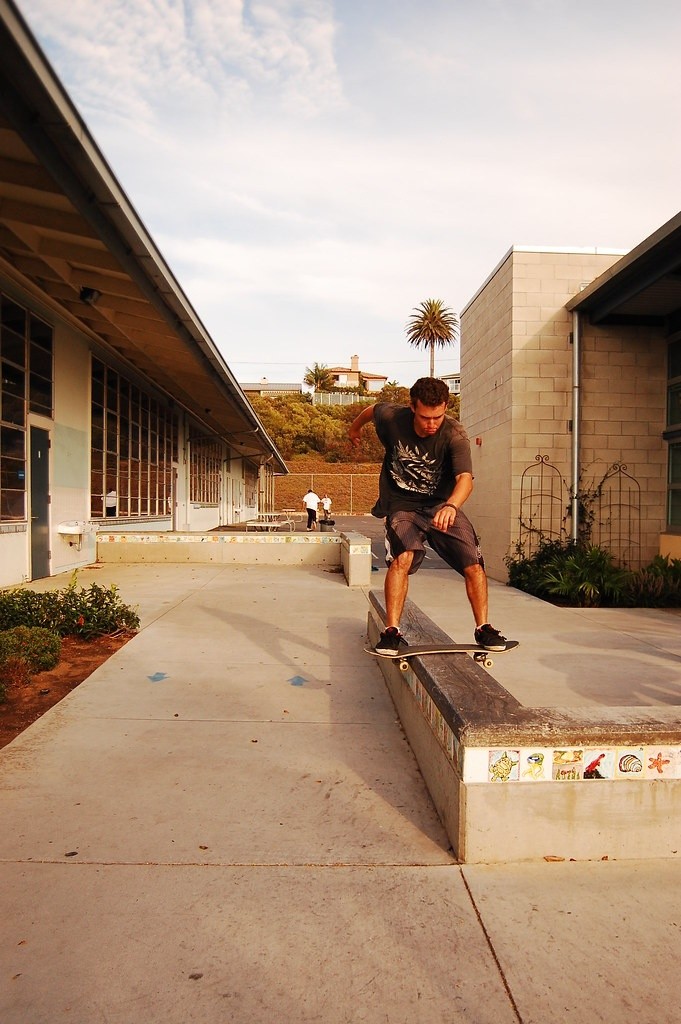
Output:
[57,519,102,536]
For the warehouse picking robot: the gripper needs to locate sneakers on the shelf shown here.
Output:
[475,623,508,651]
[374,627,408,656]
[307,528,312,532]
[312,521,316,529]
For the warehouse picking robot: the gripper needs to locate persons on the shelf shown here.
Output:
[302,489,320,532]
[320,493,332,520]
[100,488,117,517]
[347,375,507,656]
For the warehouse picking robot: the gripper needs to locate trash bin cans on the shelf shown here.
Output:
[319,519,335,532]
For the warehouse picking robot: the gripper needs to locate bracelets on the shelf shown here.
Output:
[441,503,458,512]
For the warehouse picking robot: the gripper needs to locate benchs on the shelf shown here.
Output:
[297,513,305,522]
[247,521,282,531]
[283,519,297,532]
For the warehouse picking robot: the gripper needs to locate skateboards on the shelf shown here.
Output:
[364,640,520,672]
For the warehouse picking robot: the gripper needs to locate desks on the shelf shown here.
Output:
[282,509,296,521]
[257,512,281,532]
